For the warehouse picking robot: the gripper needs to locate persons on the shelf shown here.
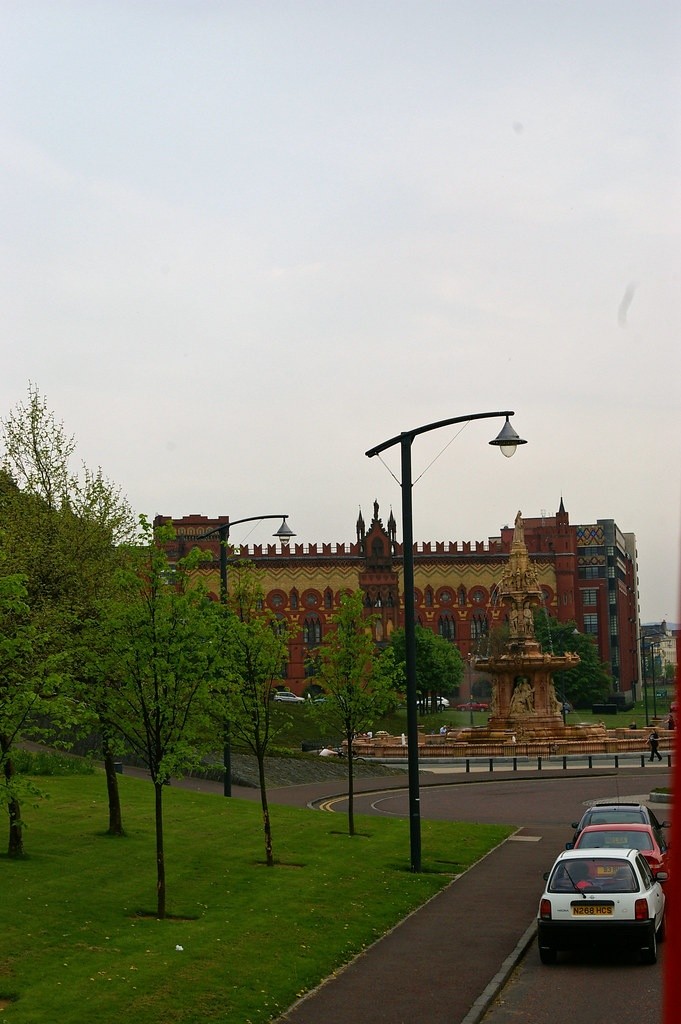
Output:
[515,725,526,736]
[490,677,562,716]
[440,725,447,734]
[598,719,606,731]
[664,715,675,730]
[629,721,636,730]
[319,745,338,756]
[509,601,533,634]
[645,730,662,762]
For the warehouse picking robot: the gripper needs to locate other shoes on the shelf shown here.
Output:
[658,758,662,761]
[648,760,653,762]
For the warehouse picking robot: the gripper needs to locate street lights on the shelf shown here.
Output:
[467,652,474,727]
[364,411,528,875]
[634,626,673,727]
[642,637,676,720]
[197,514,297,798]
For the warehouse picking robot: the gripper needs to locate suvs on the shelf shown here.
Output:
[416,696,450,711]
[273,692,306,704]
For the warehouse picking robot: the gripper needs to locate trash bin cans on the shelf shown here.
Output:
[114,761,123,774]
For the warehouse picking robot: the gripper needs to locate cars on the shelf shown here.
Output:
[565,822,667,879]
[314,696,328,703]
[571,802,671,855]
[456,699,489,713]
[537,847,668,965]
